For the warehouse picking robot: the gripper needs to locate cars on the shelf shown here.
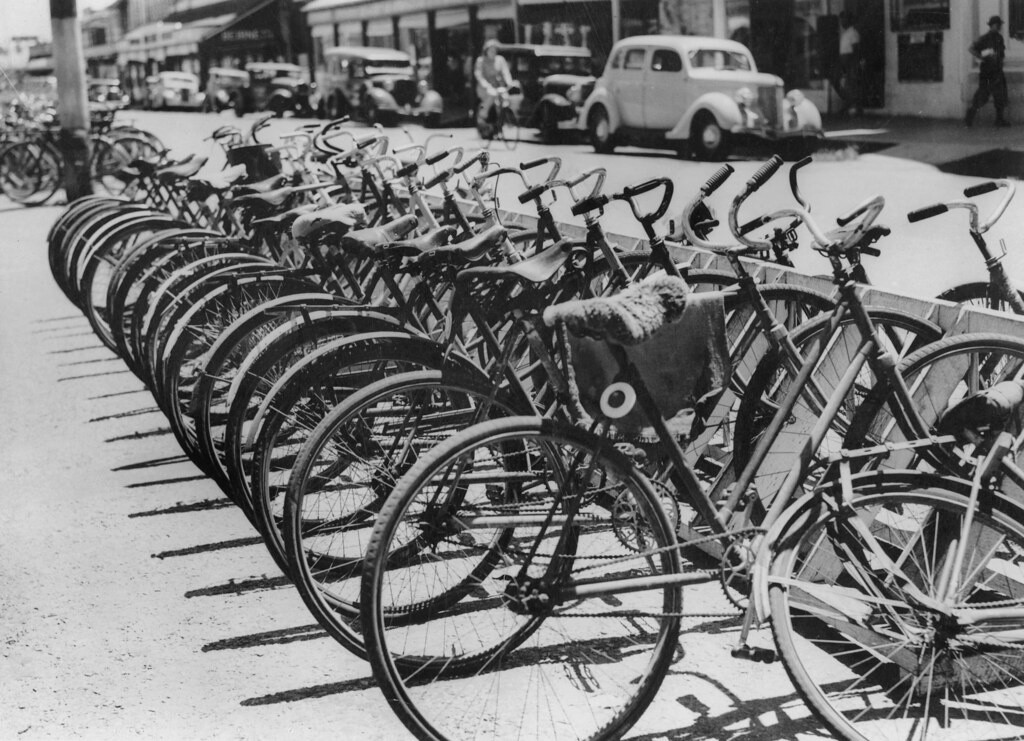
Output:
[321,46,443,131]
[497,43,599,146]
[141,71,205,111]
[576,34,825,163]
[204,66,249,114]
[229,61,316,118]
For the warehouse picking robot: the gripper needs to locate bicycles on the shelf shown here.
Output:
[476,88,521,151]
[0,83,1024,741]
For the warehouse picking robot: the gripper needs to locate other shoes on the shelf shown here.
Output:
[993,118,1011,128]
[966,107,977,126]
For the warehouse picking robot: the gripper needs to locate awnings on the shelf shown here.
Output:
[199,4,282,54]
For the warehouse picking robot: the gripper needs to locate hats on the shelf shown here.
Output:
[483,39,503,51]
[987,16,1004,26]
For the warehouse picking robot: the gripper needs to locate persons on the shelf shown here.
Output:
[964,16,1013,129]
[822,10,872,117]
[474,37,517,126]
